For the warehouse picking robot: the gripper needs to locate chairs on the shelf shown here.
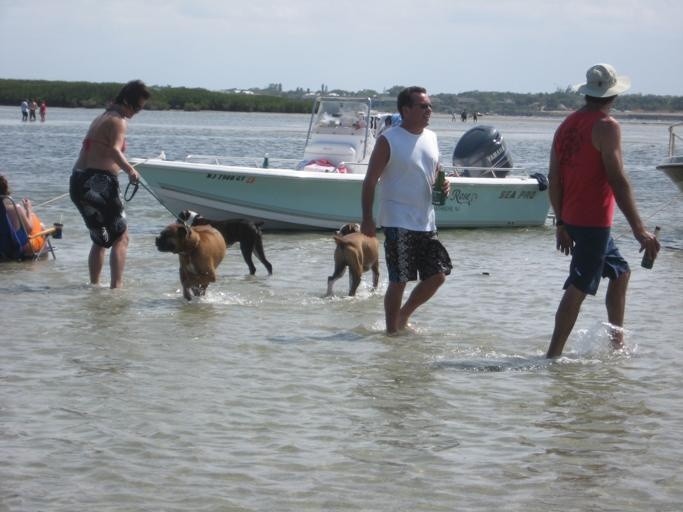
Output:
[0,193,60,265]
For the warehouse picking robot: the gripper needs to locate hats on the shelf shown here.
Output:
[573,63,636,99]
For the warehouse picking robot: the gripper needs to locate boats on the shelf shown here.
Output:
[124,91,554,235]
[652,119,683,198]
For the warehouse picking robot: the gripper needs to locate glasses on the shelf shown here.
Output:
[410,103,433,109]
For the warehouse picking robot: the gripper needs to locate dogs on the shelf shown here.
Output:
[155,222,226,301]
[176,209,272,275]
[327,222,379,296]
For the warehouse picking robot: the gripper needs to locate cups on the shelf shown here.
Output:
[51,222,62,240]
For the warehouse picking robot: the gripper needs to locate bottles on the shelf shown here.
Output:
[429,163,446,205]
[261,157,268,169]
[158,150,165,161]
[641,226,661,268]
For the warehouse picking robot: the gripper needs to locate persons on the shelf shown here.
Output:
[20,98,28,121]
[68,79,151,289]
[460,109,466,121]
[544,62,659,361]
[359,87,453,334]
[28,100,37,121]
[0,174,43,252]
[472,111,478,123]
[39,100,45,122]
[376,118,392,136]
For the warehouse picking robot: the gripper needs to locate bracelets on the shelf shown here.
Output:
[556,219,562,228]
